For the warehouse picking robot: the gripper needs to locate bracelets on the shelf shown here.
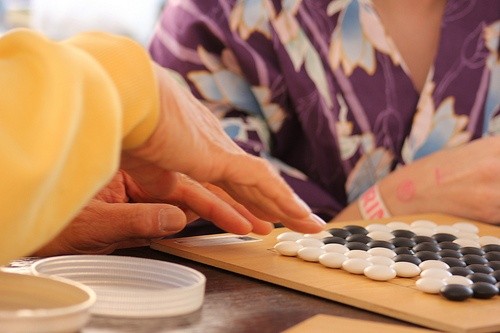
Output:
[358,182,391,221]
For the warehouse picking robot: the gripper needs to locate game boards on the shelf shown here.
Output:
[149,211,500,333]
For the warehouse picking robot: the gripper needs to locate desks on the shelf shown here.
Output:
[79,211,445,333]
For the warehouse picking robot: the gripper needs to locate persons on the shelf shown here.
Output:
[0,28,327,267]
[148,0,500,225]
